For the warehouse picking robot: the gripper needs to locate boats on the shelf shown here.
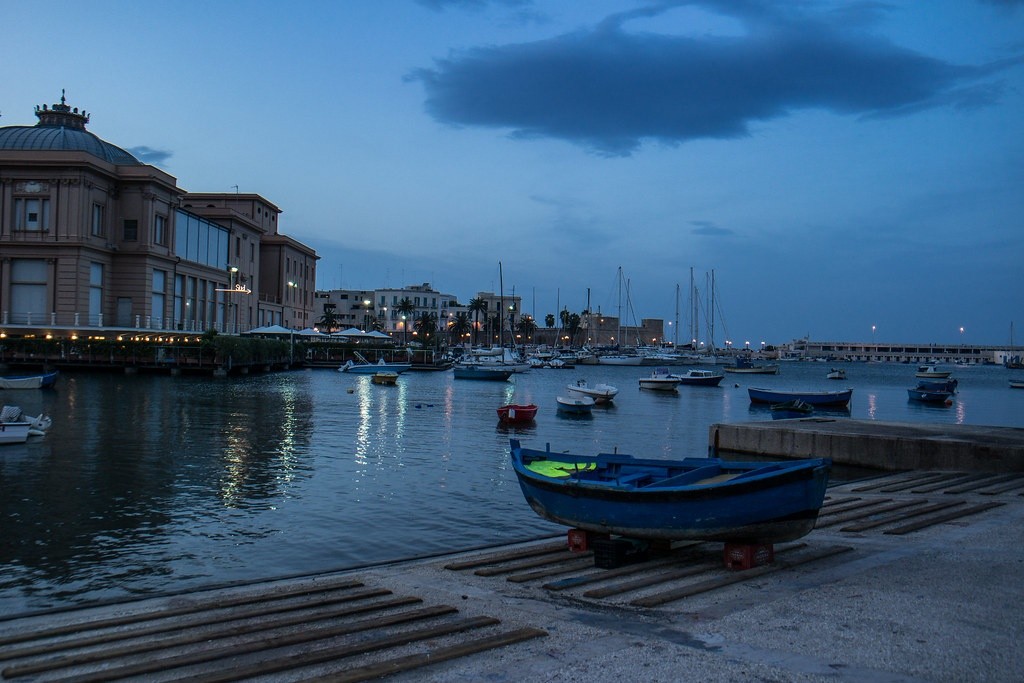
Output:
[498,402,539,423]
[1008,379,1024,388]
[748,386,854,420]
[907,360,960,404]
[0,371,57,389]
[338,351,411,384]
[556,380,619,412]
[638,355,779,390]
[508,437,833,544]
[453,347,514,381]
[0,421,31,444]
[827,368,846,380]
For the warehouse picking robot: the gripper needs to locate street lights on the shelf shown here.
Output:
[610,336,614,346]
[693,339,765,351]
[365,300,407,344]
[652,337,656,347]
[465,333,569,345]
[289,281,297,364]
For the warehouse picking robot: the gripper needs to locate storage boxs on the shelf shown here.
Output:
[568,529,610,553]
[593,539,620,569]
[724,542,774,571]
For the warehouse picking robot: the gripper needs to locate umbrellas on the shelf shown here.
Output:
[240,324,392,349]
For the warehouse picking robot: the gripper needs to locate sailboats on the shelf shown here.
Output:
[489,260,736,372]
[1005,321,1024,368]
